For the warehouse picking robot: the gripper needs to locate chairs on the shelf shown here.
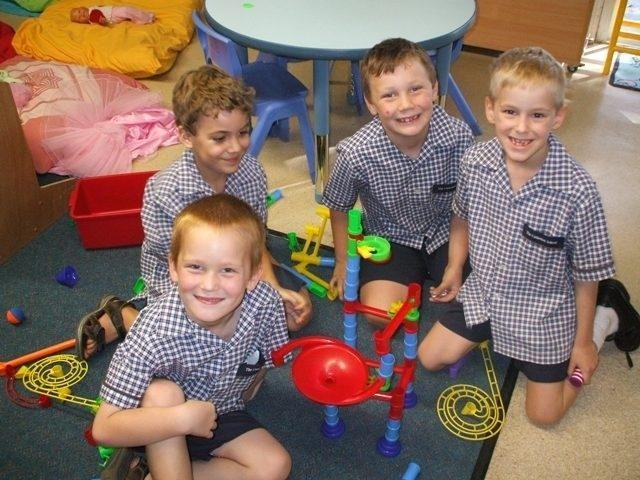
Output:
[343,26,484,135]
[190,6,319,184]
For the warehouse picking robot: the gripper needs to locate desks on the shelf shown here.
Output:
[197,1,481,204]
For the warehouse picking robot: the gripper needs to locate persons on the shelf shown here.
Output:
[70,5,158,26]
[416,46,639,428]
[77,63,312,363]
[320,39,476,326]
[92,191,293,480]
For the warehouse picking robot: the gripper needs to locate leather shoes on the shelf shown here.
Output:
[596,279,639,352]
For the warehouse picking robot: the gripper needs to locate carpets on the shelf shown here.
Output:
[2,207,520,480]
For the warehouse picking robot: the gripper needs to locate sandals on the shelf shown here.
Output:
[100,445,149,480]
[77,294,137,361]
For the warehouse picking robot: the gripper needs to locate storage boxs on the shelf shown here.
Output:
[68,166,167,250]
